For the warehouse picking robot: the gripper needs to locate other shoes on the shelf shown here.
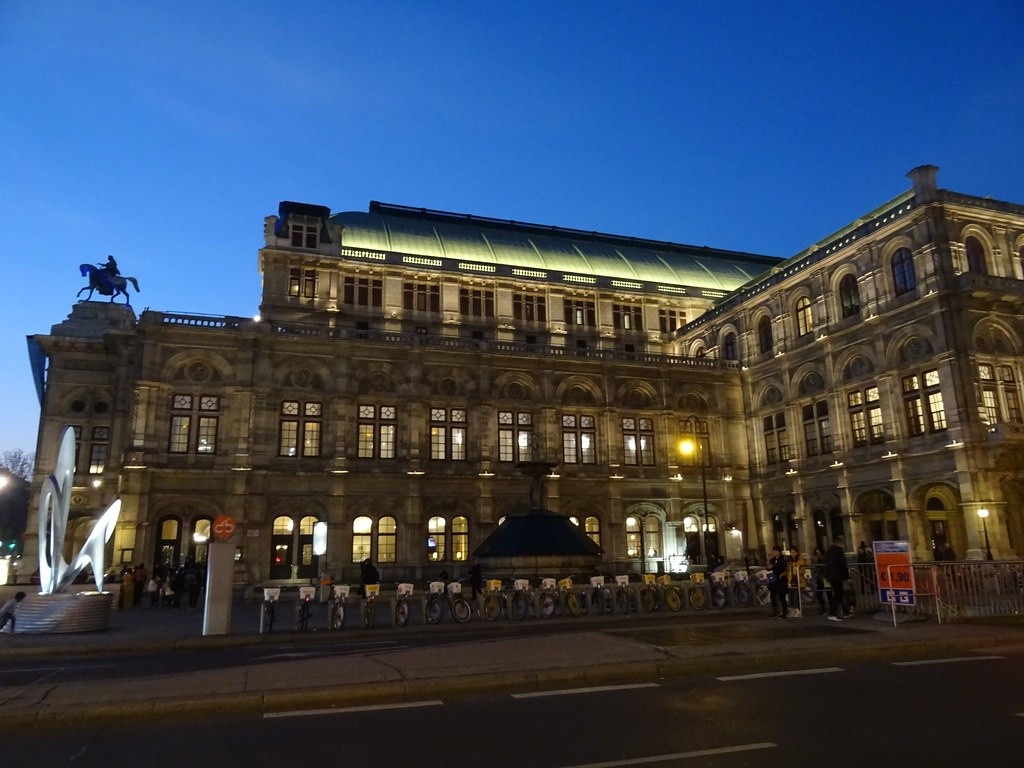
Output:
[777,613,787,618]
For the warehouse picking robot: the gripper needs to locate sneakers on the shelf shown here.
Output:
[786,608,795,617]
[793,609,802,617]
[818,610,830,616]
[841,610,853,619]
[827,615,842,621]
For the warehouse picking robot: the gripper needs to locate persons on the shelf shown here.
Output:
[359,558,380,604]
[0,592,25,636]
[119,559,206,612]
[469,561,485,600]
[766,539,955,621]
[97,255,116,290]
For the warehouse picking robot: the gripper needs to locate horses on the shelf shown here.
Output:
[76,264,140,304]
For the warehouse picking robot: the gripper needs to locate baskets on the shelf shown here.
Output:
[398,583,414,596]
[641,575,655,586]
[514,579,529,592]
[615,575,629,587]
[657,575,671,586]
[734,571,747,582]
[590,576,604,588]
[430,582,444,594]
[447,582,461,594]
[558,578,572,591]
[711,572,724,583]
[486,579,501,593]
[364,585,379,597]
[263,588,281,601]
[690,573,704,586]
[542,578,556,590]
[300,587,315,600]
[333,586,350,598]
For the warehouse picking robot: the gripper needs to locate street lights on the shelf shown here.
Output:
[678,440,715,559]
[977,506,995,560]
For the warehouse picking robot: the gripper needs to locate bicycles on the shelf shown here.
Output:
[479,577,504,622]
[296,582,320,632]
[655,574,684,611]
[615,575,639,615]
[446,571,475,623]
[687,570,708,609]
[324,581,354,632]
[536,575,560,619]
[391,580,415,627]
[557,574,584,618]
[261,584,282,633]
[509,576,533,620]
[359,579,385,628]
[642,573,659,609]
[589,574,614,616]
[423,578,447,623]
[707,567,775,608]
